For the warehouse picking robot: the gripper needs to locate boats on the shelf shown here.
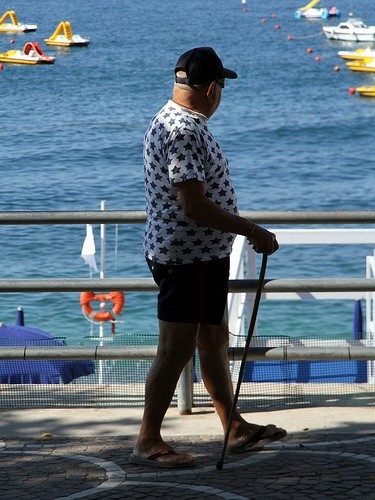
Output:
[0,41,56,65]
[322,17,375,43]
[227,227,375,384]
[337,46,375,72]
[43,21,91,47]
[0,199,125,384]
[0,10,38,33]
[294,0,340,20]
[354,84,375,97]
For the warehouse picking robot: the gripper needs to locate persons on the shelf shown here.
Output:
[128,46,287,469]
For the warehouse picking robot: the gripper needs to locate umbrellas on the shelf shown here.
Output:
[0,321,95,384]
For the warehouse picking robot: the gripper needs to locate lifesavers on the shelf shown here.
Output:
[80,291,124,321]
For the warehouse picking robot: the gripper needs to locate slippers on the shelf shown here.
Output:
[227,426,287,454]
[129,449,195,469]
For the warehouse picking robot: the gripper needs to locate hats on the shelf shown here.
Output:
[174,47,238,85]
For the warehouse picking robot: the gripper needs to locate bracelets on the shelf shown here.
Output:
[252,223,256,233]
